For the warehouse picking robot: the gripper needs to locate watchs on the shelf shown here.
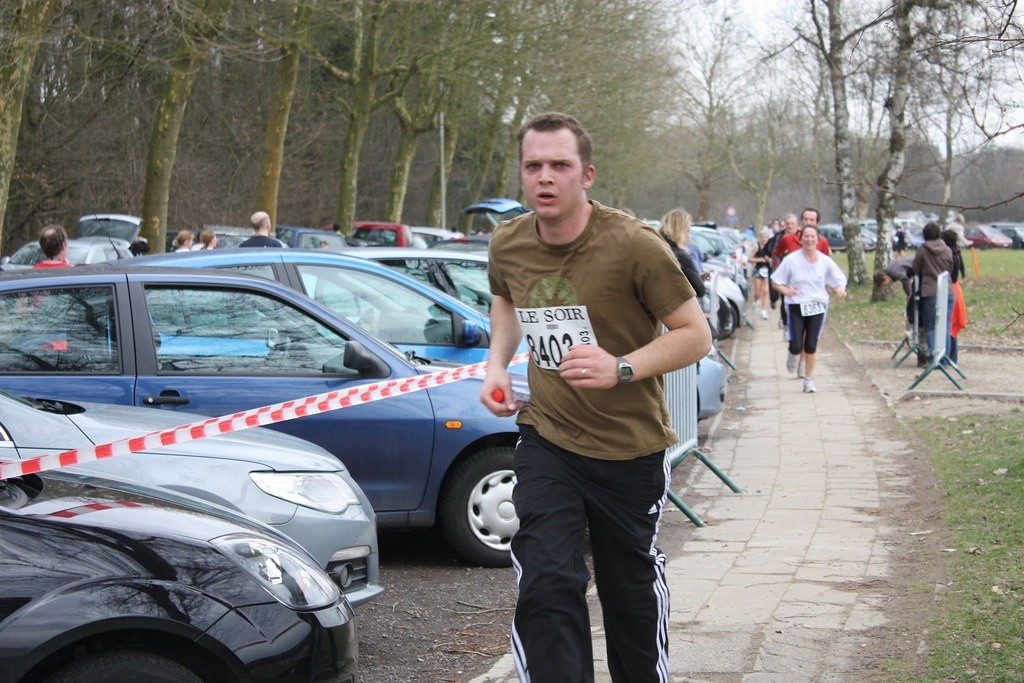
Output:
[615,356,633,386]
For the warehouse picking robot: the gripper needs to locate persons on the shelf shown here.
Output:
[239,211,285,247]
[14,223,73,317]
[895,225,906,258]
[874,222,966,369]
[131,237,150,257]
[171,228,217,253]
[746,206,849,393]
[659,207,711,298]
[480,113,715,682]
[947,213,973,281]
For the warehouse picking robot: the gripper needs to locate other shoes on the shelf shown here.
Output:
[784,331,791,342]
[798,358,805,377]
[803,380,815,393]
[787,351,798,373]
[778,318,785,329]
[771,301,776,309]
[760,310,768,320]
[752,300,759,310]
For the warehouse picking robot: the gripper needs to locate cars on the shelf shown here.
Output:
[0,263,530,569]
[0,196,774,425]
[992,221,1024,250]
[0,457,361,683]
[0,384,389,610]
[817,211,974,253]
[9,246,529,382]
[963,223,1014,250]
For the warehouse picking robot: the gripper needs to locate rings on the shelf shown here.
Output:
[581,368,587,379]
